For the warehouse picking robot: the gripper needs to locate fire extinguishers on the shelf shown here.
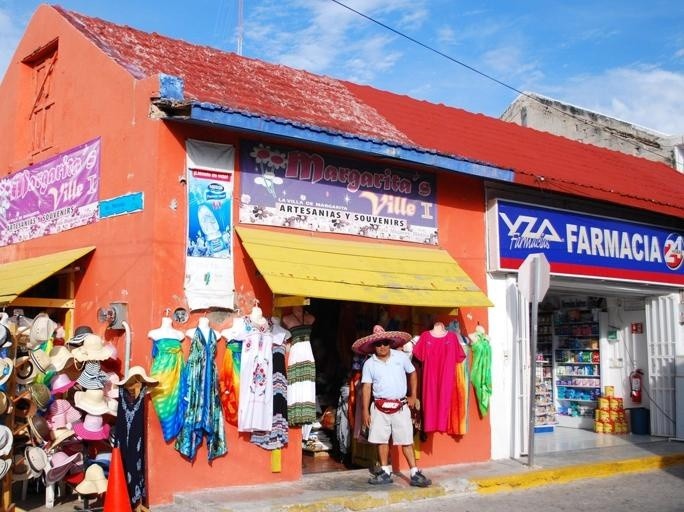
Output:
[629,369,644,403]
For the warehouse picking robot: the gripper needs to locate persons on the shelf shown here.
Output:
[412,322,492,436]
[351,325,433,486]
[148,307,316,463]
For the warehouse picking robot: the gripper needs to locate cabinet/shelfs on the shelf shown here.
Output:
[536,320,552,407]
[550,307,609,429]
[532,360,553,434]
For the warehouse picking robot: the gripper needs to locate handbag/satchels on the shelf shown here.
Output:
[374,399,403,414]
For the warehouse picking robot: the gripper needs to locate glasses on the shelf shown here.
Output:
[373,341,388,347]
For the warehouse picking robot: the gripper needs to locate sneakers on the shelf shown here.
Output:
[410,470,432,487]
[368,470,393,485]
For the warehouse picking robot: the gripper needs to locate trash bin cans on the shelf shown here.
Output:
[630,408,650,435]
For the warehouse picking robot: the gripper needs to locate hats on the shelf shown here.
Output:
[0,311,118,495]
[117,365,159,390]
[351,325,413,355]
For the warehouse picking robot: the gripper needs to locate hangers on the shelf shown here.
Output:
[114,364,159,387]
